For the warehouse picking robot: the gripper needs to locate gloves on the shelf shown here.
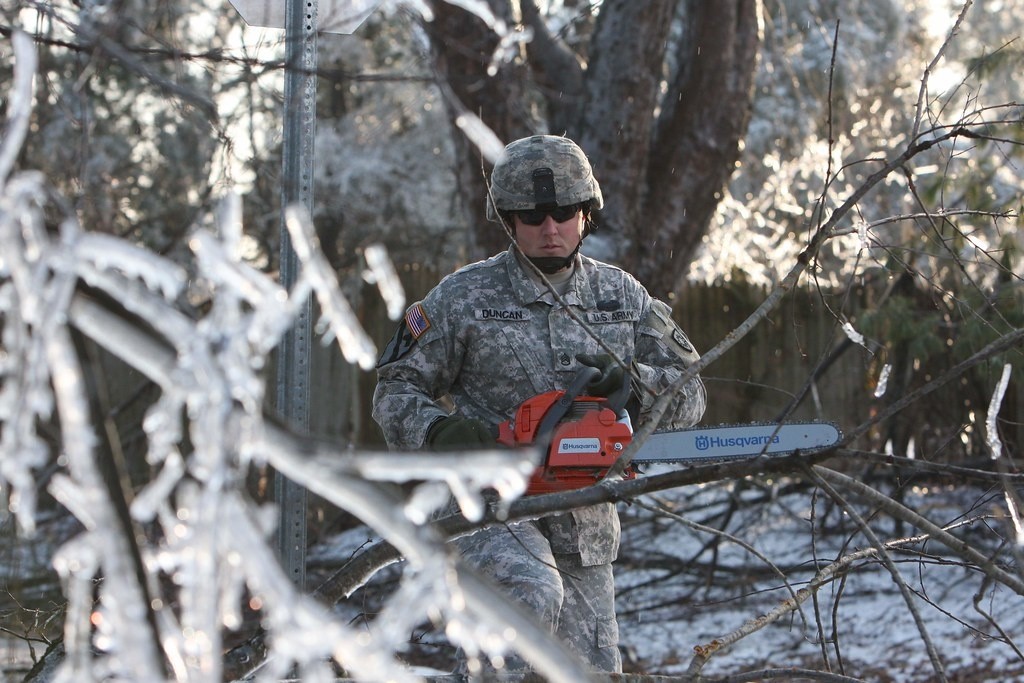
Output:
[575,352,641,400]
[425,414,499,452]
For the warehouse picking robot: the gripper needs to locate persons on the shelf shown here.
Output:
[370,134,707,683]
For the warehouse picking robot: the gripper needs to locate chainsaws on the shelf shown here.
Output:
[489,368,847,498]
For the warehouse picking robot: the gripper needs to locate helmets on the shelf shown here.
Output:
[485,134,604,224]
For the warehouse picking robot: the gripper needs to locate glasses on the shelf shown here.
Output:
[501,200,591,225]
